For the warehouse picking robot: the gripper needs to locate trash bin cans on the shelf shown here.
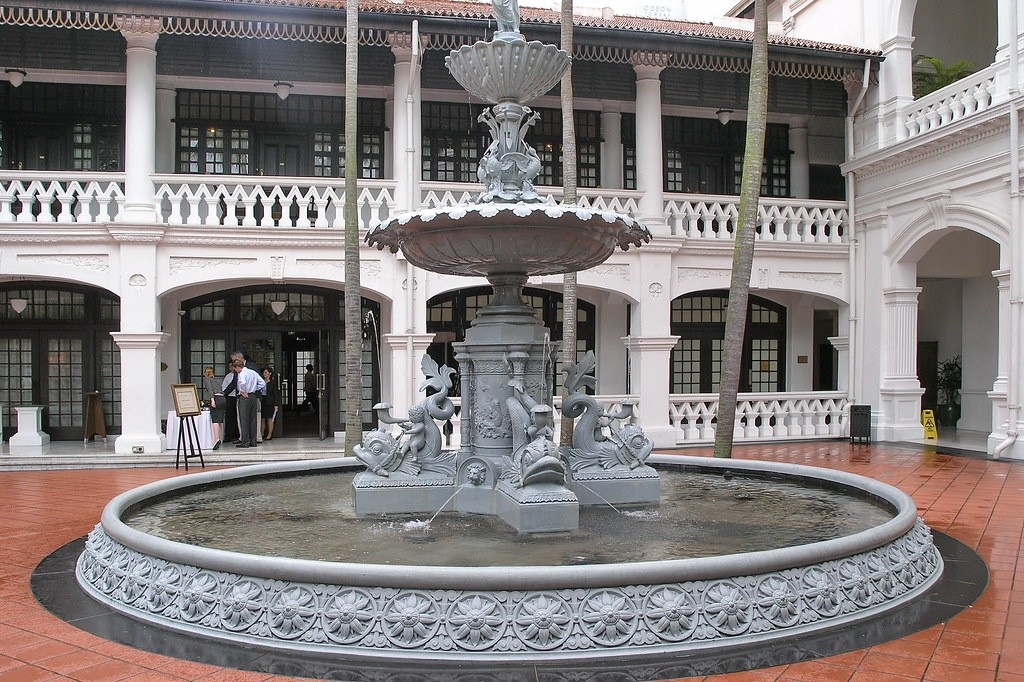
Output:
[851,403,872,444]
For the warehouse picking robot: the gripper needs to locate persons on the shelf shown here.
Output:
[294,364,319,416]
[204,351,280,450]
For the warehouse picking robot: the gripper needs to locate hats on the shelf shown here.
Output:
[306,364,313,370]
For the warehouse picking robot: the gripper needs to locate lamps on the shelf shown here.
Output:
[5,62,27,88]
[8,297,30,313]
[715,107,734,126]
[273,80,294,100]
[269,300,288,315]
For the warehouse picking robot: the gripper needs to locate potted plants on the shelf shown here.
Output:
[937,354,961,426]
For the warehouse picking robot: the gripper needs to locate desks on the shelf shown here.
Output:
[166,411,262,450]
[78,391,110,443]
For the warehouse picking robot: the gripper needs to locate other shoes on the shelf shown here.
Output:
[213,440,221,450]
[233,439,262,448]
[262,436,272,441]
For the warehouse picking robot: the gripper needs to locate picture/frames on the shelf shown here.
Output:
[171,383,202,417]
